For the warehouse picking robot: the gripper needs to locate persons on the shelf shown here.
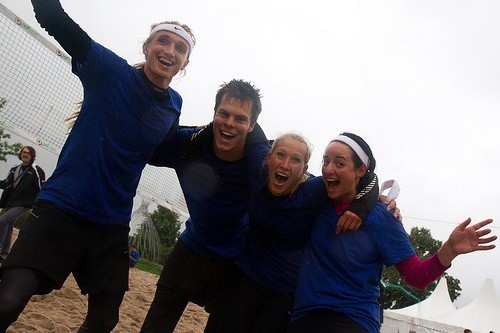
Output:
[0,146,45,267]
[291,133,497,333]
[0,0,196,333]
[205,120,379,333]
[128,245,140,268]
[139,79,402,333]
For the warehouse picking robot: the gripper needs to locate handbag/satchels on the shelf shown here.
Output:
[0,184,13,208]
